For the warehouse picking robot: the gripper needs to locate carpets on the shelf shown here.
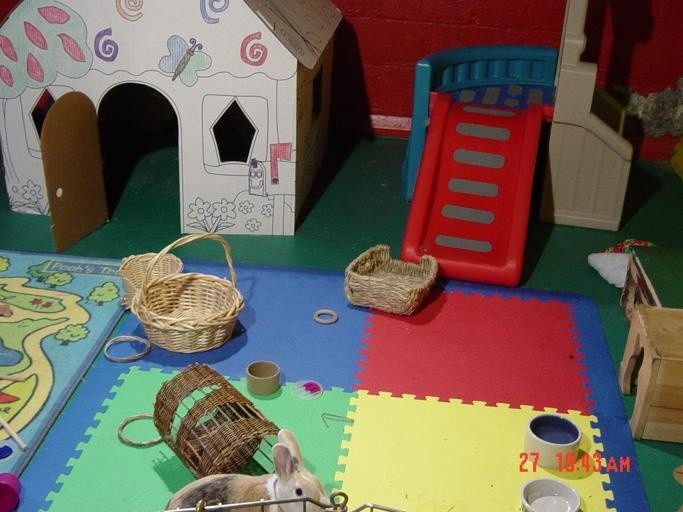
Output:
[0,246,663,512]
[0,249,126,487]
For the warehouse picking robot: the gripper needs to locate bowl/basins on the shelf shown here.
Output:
[524,414,580,467]
[521,479,579,511]
[246,360,280,395]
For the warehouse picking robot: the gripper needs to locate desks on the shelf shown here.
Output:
[618,247,683,325]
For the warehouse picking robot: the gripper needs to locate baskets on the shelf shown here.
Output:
[131,232,244,354]
[118,252,183,311]
[343,244,437,316]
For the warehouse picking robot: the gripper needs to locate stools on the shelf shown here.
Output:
[618,301,683,443]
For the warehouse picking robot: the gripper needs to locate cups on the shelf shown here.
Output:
[0,471,20,512]
